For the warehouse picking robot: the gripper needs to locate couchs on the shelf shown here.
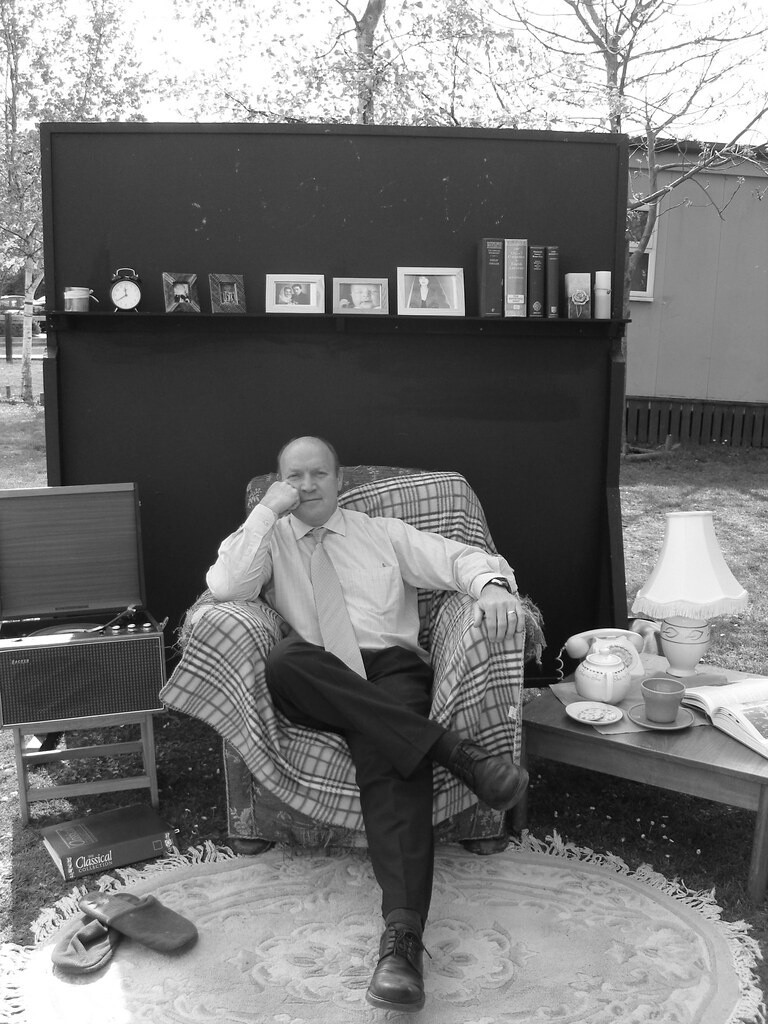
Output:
[159,467,545,855]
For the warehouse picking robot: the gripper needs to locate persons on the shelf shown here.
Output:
[204,435,529,1008]
[278,284,309,304]
[410,275,440,307]
[340,283,380,310]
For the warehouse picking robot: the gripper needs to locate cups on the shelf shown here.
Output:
[640,678,686,723]
[64,286,90,312]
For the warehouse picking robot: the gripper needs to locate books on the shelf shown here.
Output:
[681,674,768,759]
[504,238,529,316]
[528,245,547,317]
[39,801,176,881]
[476,238,503,317]
[547,245,561,317]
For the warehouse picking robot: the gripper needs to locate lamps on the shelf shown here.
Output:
[631,512,750,677]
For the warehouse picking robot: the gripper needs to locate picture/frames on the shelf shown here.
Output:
[396,268,466,316]
[332,278,391,314]
[266,274,325,313]
[208,274,247,314]
[162,272,203,312]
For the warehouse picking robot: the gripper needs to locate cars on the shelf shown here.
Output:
[0,295,46,314]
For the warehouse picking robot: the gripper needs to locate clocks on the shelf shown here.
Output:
[109,268,141,312]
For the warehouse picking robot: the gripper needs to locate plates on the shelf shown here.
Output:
[628,703,694,730]
[566,701,624,726]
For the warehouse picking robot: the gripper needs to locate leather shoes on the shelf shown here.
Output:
[447,738,531,811]
[364,924,432,1012]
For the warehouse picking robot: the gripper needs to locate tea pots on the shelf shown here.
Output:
[575,647,631,706]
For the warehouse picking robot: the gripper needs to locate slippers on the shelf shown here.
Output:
[79,889,197,955]
[52,892,136,974]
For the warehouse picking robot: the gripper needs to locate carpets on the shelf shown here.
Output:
[1,820,767,1024]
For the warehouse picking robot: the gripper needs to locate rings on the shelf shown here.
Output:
[507,610,516,614]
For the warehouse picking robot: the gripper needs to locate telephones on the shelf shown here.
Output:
[565,628,645,678]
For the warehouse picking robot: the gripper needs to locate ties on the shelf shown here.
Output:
[307,527,367,681]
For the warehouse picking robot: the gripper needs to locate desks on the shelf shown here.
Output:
[520,651,768,905]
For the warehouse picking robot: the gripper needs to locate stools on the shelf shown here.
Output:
[16,712,159,825]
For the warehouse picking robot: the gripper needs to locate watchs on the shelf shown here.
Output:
[490,578,508,588]
